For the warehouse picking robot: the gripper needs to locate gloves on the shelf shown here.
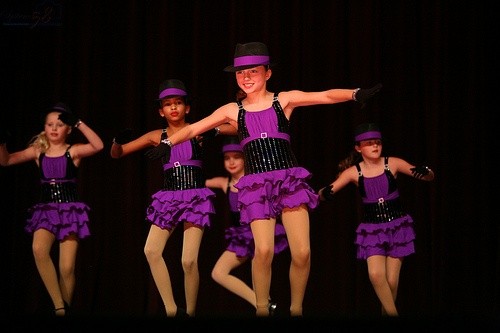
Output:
[58,112,79,126]
[146,144,171,163]
[322,185,334,200]
[409,166,430,179]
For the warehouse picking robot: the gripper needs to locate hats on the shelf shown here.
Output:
[223,42,272,71]
[48,101,71,111]
[354,122,382,140]
[155,79,187,100]
[220,134,243,154]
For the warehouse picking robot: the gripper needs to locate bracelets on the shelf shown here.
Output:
[75,121,83,128]
[162,139,173,147]
[352,88,360,101]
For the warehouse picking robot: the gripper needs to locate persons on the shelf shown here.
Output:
[202,133,289,310]
[149,42,378,317]
[110,77,238,316]
[0,102,104,315]
[318,126,435,317]
